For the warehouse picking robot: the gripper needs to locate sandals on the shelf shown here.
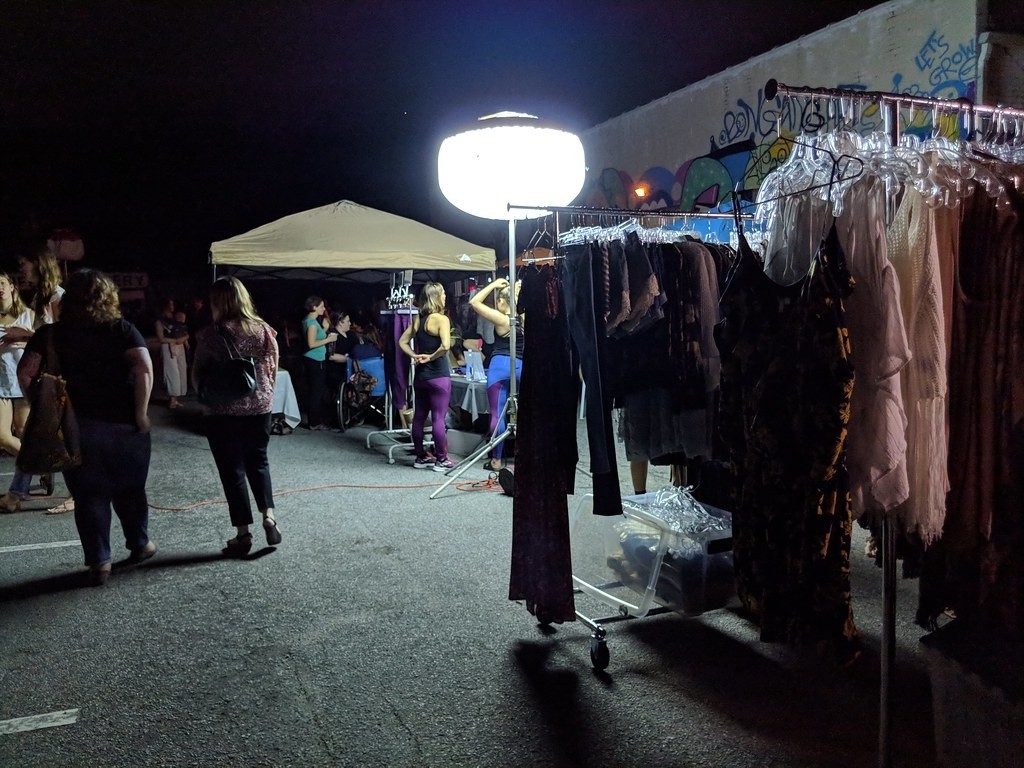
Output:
[0,497,20,513]
[48,501,77,514]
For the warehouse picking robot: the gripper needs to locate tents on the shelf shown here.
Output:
[207,201,497,313]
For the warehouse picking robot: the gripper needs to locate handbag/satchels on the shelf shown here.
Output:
[15,372,81,474]
[196,331,258,405]
[346,370,378,408]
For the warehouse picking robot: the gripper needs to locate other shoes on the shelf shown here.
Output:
[169,399,184,409]
[309,423,330,430]
[87,564,111,583]
[131,541,156,565]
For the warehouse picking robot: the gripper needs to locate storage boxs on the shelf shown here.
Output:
[569,492,739,618]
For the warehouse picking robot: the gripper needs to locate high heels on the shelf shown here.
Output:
[226,532,252,553]
[263,517,282,545]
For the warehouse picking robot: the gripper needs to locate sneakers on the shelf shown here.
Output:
[433,460,457,471]
[413,456,437,469]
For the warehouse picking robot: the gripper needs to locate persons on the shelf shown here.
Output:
[0,272,55,495]
[399,282,459,471]
[192,277,284,553]
[0,252,73,514]
[304,297,352,431]
[16,269,158,584]
[469,278,525,470]
[164,313,190,357]
[156,298,189,408]
[352,325,383,360]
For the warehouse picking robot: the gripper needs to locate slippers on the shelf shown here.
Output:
[484,460,502,472]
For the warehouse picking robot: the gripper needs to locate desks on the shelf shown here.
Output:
[450,373,491,435]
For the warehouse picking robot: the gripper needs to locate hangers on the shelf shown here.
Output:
[521,82,1024,263]
[383,294,421,312]
